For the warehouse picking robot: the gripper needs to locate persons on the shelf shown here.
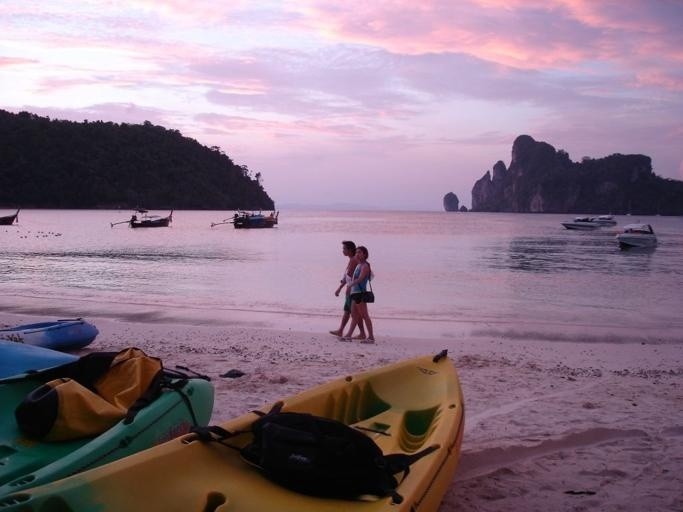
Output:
[328,239,366,340]
[338,245,375,344]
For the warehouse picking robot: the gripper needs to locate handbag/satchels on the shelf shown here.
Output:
[363,292,374,302]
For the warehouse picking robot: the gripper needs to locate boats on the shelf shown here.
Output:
[232,211,279,229]
[0,207,22,226]
[615,223,657,249]
[0,347,216,500]
[0,349,466,512]
[559,214,616,230]
[0,317,99,351]
[1,339,80,380]
[130,209,174,228]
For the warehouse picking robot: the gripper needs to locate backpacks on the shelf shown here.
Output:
[14,347,212,444]
[199,400,439,504]
